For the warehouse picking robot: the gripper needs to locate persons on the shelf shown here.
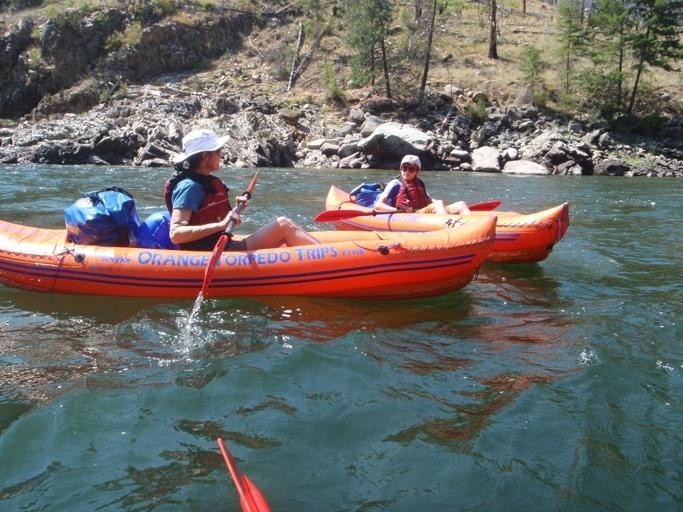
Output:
[163,127,325,253]
[370,153,471,215]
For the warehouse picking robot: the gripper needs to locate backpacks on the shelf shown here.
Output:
[61,187,142,247]
[349,178,387,208]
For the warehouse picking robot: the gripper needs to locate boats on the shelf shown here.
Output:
[0,214,499,299]
[325,185,569,263]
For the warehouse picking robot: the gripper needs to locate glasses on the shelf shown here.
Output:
[400,165,418,173]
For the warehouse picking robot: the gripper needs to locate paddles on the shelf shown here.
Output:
[217,438,271,512]
[315,201,502,222]
[202,168,261,296]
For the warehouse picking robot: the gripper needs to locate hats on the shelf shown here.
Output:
[170,127,230,165]
[399,154,422,171]
